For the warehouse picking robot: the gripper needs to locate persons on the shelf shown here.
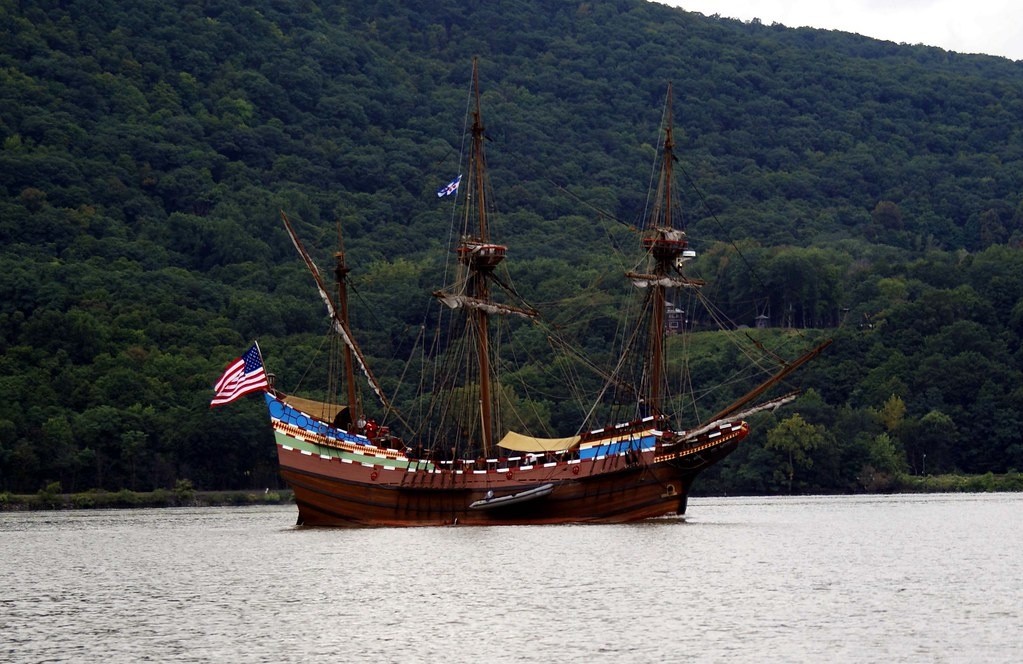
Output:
[523,451,537,466]
[398,436,450,471]
[357,414,392,449]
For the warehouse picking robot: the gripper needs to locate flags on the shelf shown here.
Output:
[209,341,271,410]
[437,174,462,198]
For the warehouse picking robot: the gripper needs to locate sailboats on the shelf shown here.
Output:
[258,57,839,529]
